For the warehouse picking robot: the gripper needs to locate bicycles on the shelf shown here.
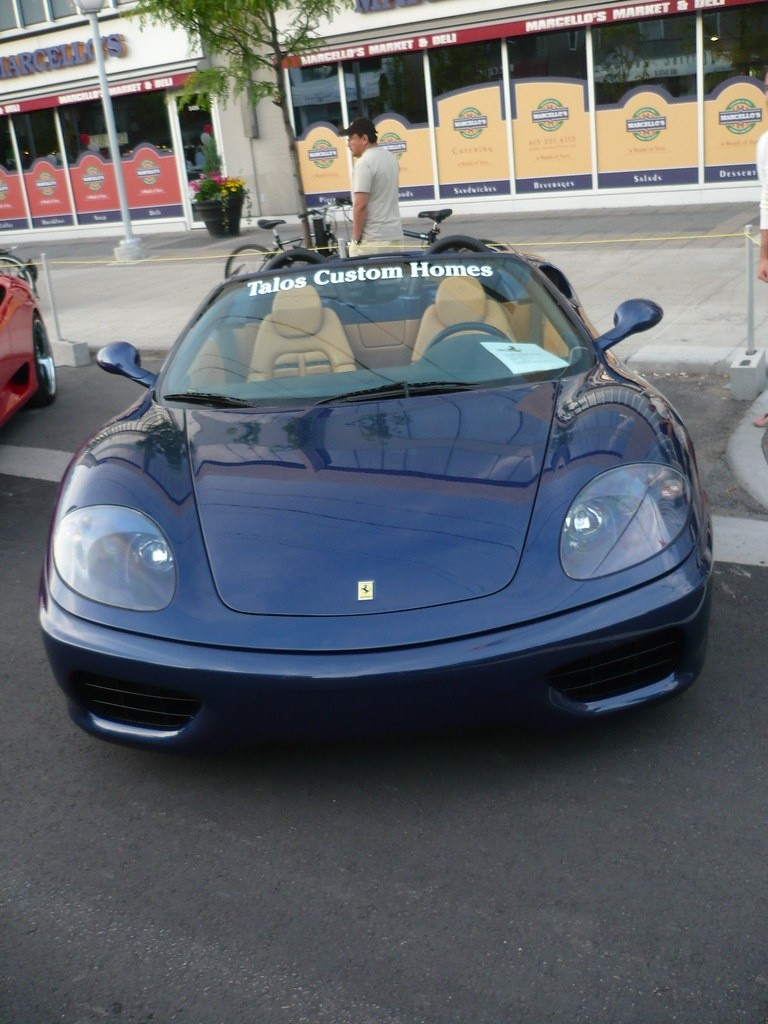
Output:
[336,197,508,250]
[0,249,41,299]
[220,199,345,281]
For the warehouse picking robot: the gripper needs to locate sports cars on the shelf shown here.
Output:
[0,275,57,440]
[35,235,712,756]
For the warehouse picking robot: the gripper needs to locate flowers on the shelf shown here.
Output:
[189,134,254,234]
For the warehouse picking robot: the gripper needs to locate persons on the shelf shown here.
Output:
[753,127,768,427]
[340,118,404,257]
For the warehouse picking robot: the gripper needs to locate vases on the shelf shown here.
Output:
[192,196,244,238]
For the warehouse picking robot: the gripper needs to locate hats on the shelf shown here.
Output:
[337,117,377,136]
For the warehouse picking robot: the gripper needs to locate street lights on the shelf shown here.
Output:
[77,0,145,264]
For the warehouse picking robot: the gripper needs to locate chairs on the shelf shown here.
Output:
[248,285,356,382]
[410,276,517,364]
